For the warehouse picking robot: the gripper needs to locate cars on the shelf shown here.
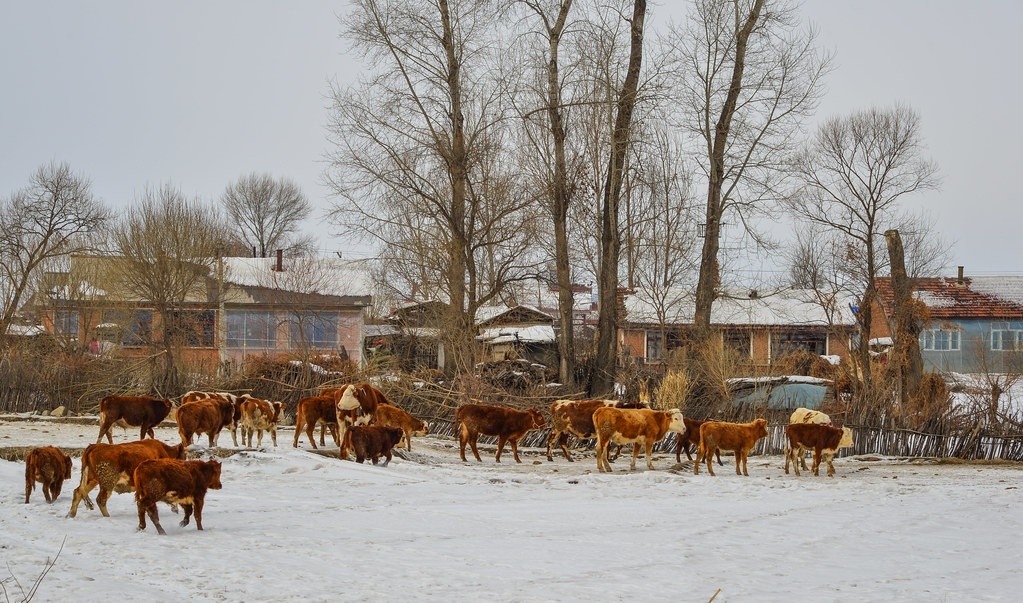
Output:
[703,373,833,417]
[255,360,346,387]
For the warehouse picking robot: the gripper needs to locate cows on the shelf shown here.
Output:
[24,445,73,505]
[453,398,769,477]
[174,390,288,449]
[65,438,223,536]
[783,407,855,479]
[95,395,173,445]
[292,382,430,467]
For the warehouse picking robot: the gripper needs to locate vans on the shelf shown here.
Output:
[474,358,532,387]
